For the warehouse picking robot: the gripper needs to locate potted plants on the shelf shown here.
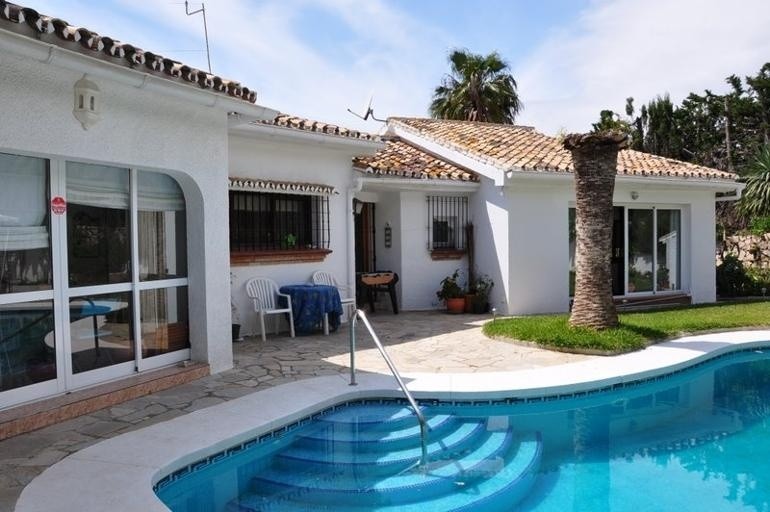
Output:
[434,267,497,315]
[228,272,243,341]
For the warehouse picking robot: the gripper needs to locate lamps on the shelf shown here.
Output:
[70,75,103,132]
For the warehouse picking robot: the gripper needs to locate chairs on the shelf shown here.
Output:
[245,277,297,342]
[310,269,358,336]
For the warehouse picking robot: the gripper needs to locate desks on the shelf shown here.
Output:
[273,284,344,337]
[1,299,128,314]
